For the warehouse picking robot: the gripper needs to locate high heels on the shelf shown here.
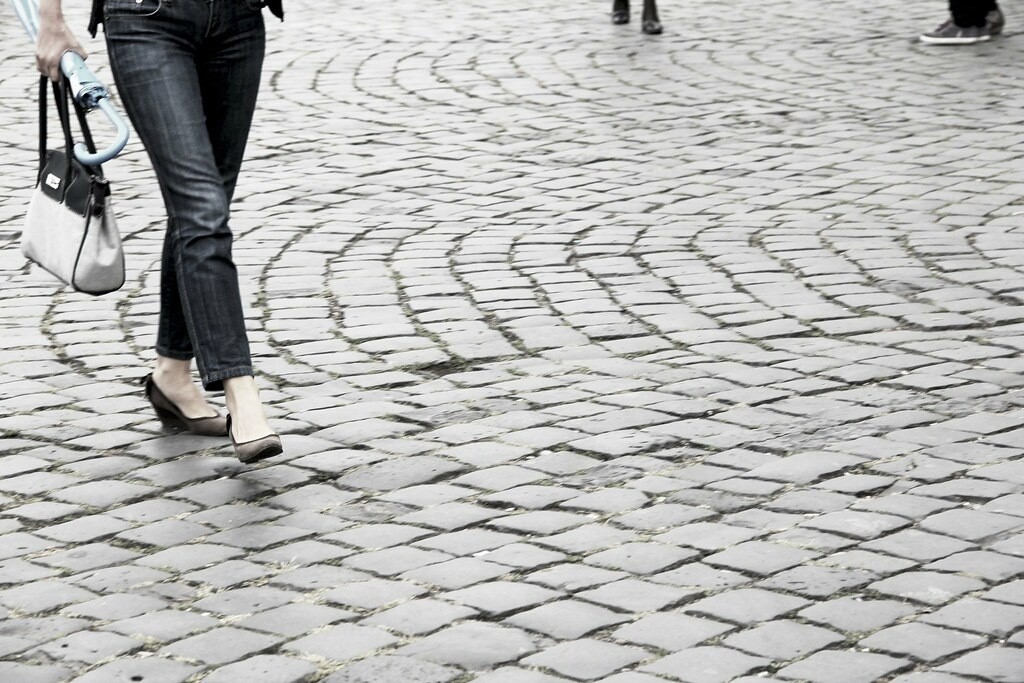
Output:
[225,412,284,465]
[139,372,230,437]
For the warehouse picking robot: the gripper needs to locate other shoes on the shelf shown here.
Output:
[612,0,631,25]
[642,12,663,35]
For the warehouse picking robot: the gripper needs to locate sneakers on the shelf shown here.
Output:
[919,7,1005,45]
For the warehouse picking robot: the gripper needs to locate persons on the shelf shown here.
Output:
[921,0,1004,44]
[612,0,661,33]
[34,0,283,462]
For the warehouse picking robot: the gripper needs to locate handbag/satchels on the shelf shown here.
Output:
[20,68,126,297]
